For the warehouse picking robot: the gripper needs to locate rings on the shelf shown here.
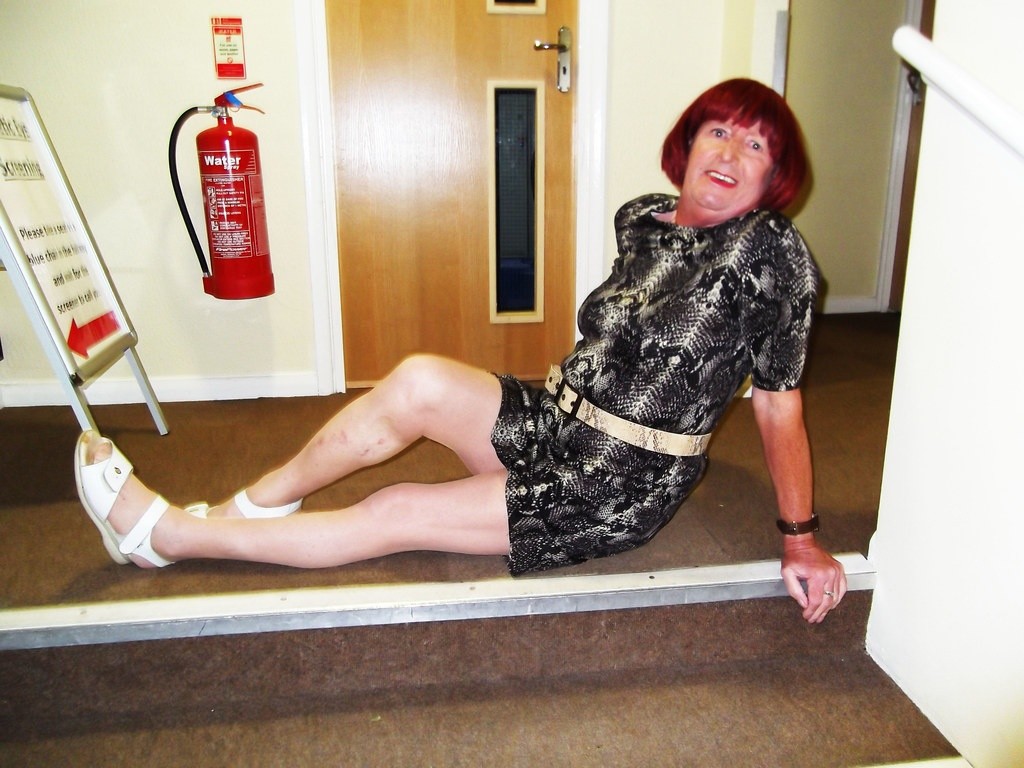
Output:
[824,590,834,596]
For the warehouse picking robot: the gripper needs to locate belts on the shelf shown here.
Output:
[544,364,714,455]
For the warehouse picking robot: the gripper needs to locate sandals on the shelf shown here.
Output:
[180,490,303,519]
[73,429,175,567]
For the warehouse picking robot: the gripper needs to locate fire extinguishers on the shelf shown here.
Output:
[166,82,279,303]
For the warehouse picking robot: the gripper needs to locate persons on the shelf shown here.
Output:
[73,78,848,626]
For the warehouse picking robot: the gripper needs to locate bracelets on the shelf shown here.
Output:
[775,513,820,537]
